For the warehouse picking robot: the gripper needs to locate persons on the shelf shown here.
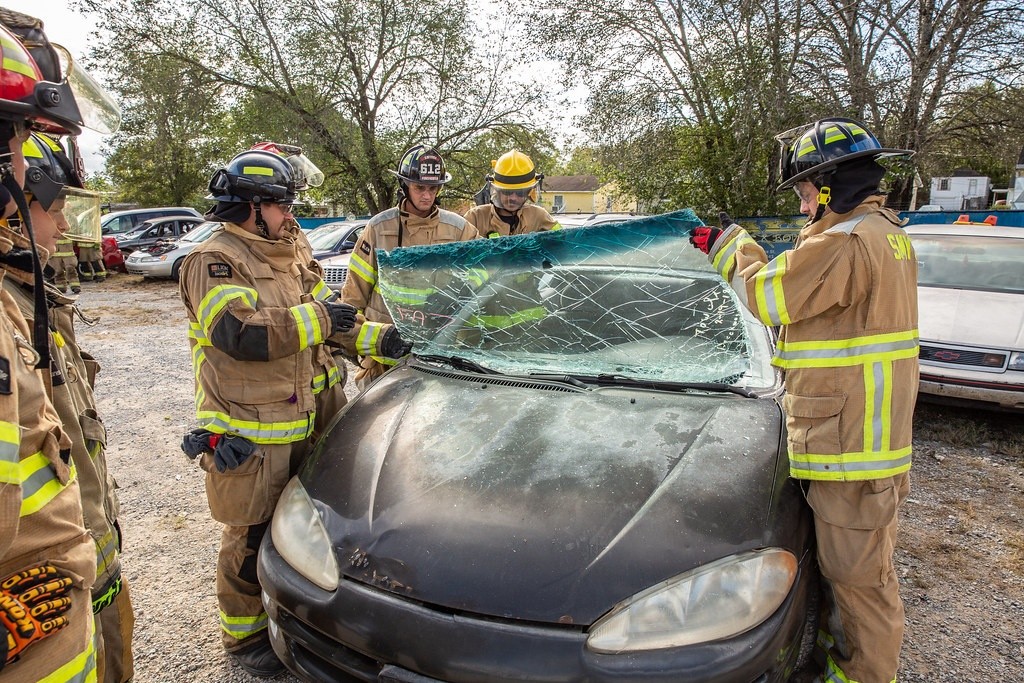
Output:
[688,117,921,683]
[0,11,134,683]
[340,145,495,391]
[463,150,563,353]
[180,142,410,675]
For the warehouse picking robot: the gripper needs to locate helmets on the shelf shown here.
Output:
[395,145,452,185]
[21,129,99,211]
[486,150,544,189]
[204,150,296,203]
[251,141,309,191]
[776,117,916,193]
[0,7,123,135]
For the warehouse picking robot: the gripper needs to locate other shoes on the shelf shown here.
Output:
[229,641,286,676]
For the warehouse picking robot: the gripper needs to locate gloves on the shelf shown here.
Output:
[689,212,733,255]
[181,428,253,474]
[385,331,414,358]
[325,290,357,332]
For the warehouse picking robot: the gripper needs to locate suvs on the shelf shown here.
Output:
[550,210,657,229]
[100,208,204,235]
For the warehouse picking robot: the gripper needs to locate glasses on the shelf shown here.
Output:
[799,186,815,203]
[501,189,531,197]
[278,202,291,215]
[404,180,440,195]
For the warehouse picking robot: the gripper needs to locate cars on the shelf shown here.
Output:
[124,221,223,285]
[901,224,1024,416]
[104,216,207,274]
[303,218,370,300]
[253,261,821,683]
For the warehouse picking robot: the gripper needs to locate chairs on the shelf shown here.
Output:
[977,261,1024,288]
[539,283,604,339]
[659,279,739,339]
[916,254,950,282]
